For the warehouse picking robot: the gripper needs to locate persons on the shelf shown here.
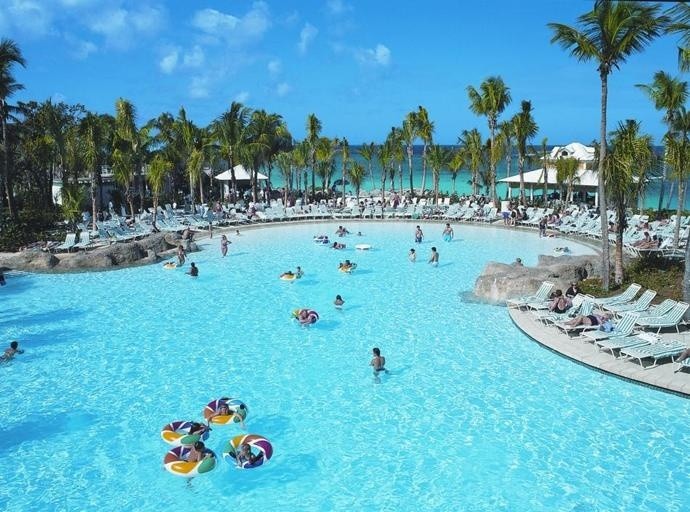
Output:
[340,260,352,270]
[333,242,342,249]
[335,295,343,305]
[631,221,652,237]
[335,226,346,237]
[284,266,302,278]
[370,348,391,384]
[548,290,566,313]
[0,341,24,364]
[81,208,91,229]
[125,216,133,226]
[624,232,663,250]
[409,249,416,261]
[206,402,246,427]
[184,422,212,434]
[676,347,690,362]
[566,280,581,299]
[517,258,523,265]
[416,226,424,242]
[97,207,109,222]
[428,247,438,262]
[186,440,211,490]
[442,223,454,242]
[236,230,240,235]
[186,233,192,242]
[221,235,231,256]
[199,182,346,223]
[559,313,609,325]
[359,189,486,218]
[236,443,257,467]
[319,235,328,243]
[186,262,198,276]
[498,189,601,238]
[177,245,186,264]
[176,200,182,209]
[607,222,616,232]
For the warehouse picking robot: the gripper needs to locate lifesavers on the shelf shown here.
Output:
[161,397,273,476]
[339,262,358,273]
[291,308,318,325]
[280,273,295,280]
[314,234,346,248]
[163,261,176,268]
[356,244,372,251]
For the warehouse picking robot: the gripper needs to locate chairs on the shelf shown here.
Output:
[505,280,689,374]
[38,193,689,263]
